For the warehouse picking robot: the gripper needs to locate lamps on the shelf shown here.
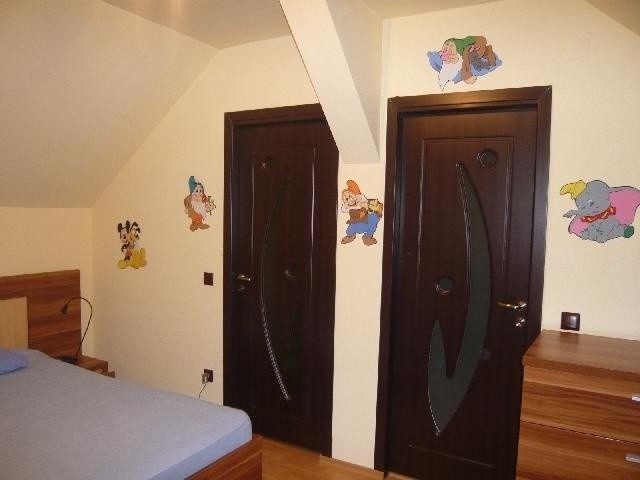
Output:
[57,297,93,362]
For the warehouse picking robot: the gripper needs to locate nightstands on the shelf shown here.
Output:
[72,354,115,377]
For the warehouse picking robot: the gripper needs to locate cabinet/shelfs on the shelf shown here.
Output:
[515,330,640,478]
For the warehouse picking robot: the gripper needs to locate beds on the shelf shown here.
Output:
[0,294,261,480]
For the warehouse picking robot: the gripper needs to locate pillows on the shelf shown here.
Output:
[0,345,28,374]
